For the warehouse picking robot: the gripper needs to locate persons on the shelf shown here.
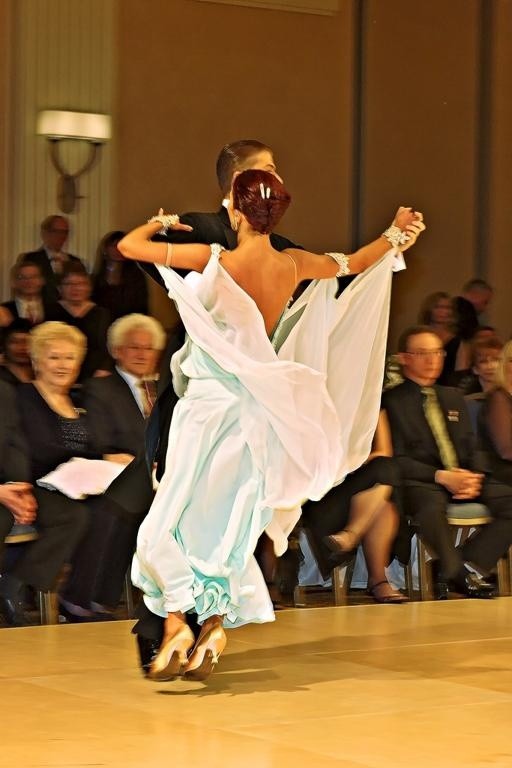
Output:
[1,215,185,626]
[117,141,425,682]
[256,277,511,609]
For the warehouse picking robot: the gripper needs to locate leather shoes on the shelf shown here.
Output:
[136,633,177,682]
[1,594,118,627]
[430,565,495,601]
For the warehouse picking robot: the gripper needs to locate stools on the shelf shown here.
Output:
[416,500,512,599]
[3,521,63,626]
[290,522,354,604]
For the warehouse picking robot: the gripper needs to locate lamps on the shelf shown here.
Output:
[33,104,115,217]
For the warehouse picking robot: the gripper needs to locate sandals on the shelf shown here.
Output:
[324,529,357,556]
[365,579,411,603]
[266,579,285,610]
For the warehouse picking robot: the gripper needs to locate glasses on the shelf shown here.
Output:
[16,224,90,289]
[398,348,446,358]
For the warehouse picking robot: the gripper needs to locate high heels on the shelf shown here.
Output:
[149,624,228,683]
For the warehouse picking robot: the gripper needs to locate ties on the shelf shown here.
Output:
[135,381,152,419]
[421,386,460,469]
